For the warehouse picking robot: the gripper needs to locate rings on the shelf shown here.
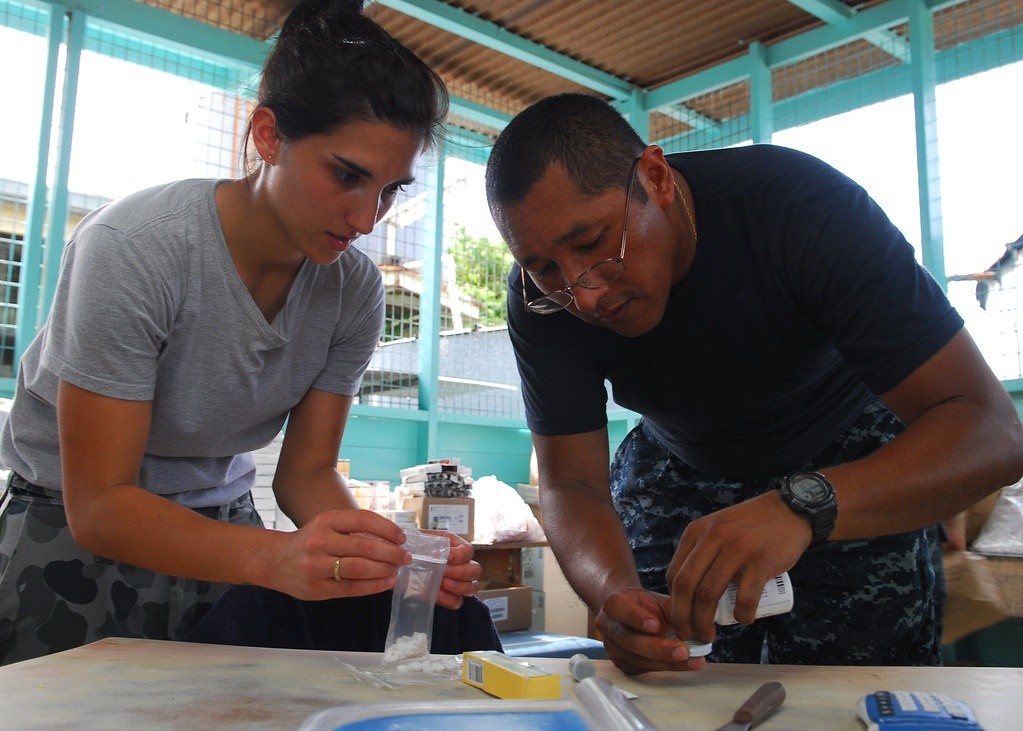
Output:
[335,557,342,582]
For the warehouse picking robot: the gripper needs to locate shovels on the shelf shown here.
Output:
[715,681,786,731]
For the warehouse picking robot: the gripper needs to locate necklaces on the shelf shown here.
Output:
[674,179,697,243]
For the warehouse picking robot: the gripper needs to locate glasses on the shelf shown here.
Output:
[520,155,640,316]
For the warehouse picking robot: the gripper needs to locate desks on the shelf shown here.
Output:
[0,636,1023,731]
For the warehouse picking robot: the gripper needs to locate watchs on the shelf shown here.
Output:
[770,469,838,551]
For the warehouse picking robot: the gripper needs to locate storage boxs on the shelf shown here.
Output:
[402,495,475,542]
[460,649,561,700]
[474,583,532,632]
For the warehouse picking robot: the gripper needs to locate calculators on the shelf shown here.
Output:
[854,689,983,731]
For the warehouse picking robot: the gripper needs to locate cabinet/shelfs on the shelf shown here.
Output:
[472,540,550,590]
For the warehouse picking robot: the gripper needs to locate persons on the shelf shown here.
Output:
[1,0,500,667]
[484,94,1023,679]
